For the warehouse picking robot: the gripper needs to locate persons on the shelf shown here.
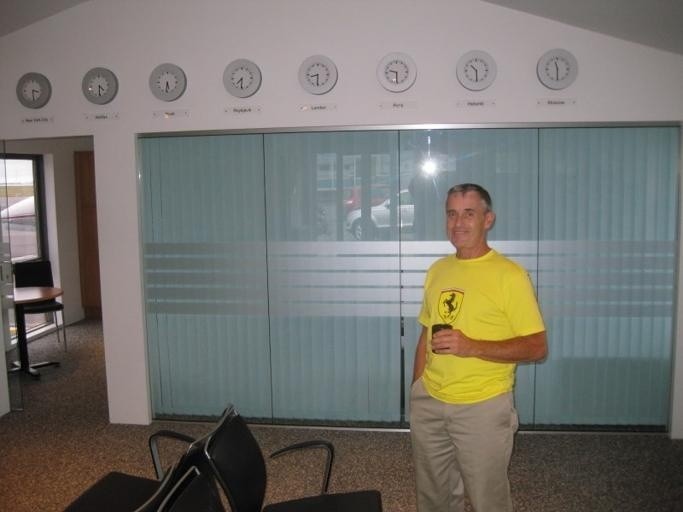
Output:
[409,183,550,511]
[407,151,457,239]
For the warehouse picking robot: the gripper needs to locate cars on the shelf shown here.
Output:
[316,184,416,240]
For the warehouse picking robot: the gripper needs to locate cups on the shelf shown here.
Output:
[432,323,453,355]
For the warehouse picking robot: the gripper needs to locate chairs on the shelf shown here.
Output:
[204,407,387,511]
[61,404,232,512]
[12,259,64,342]
[158,466,228,512]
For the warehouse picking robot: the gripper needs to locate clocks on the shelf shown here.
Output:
[149,63,188,103]
[81,66,118,107]
[374,51,418,93]
[297,53,340,99]
[15,70,55,111]
[454,51,498,91]
[221,56,264,100]
[535,49,579,90]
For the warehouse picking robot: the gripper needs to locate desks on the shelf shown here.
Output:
[3,287,65,380]
